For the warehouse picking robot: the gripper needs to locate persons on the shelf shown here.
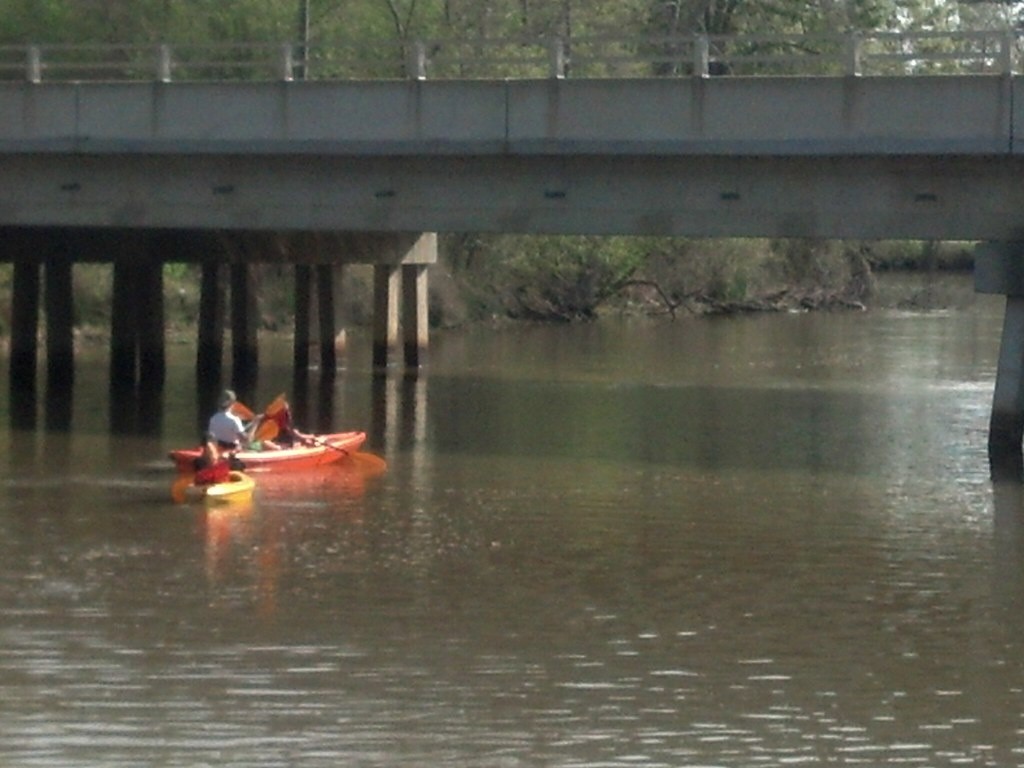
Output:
[192,389,317,485]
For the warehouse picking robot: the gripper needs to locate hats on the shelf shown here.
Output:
[218,390,236,406]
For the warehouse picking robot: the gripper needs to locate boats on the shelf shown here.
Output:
[166,432,367,474]
[171,470,256,506]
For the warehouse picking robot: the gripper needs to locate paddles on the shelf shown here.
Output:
[230,401,284,431]
[230,419,279,455]
[306,435,388,470]
